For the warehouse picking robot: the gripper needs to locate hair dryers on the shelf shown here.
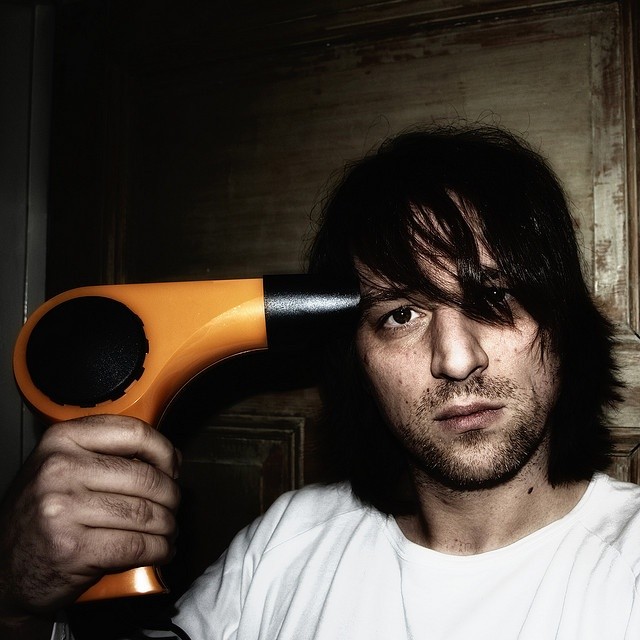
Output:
[13,276,362,606]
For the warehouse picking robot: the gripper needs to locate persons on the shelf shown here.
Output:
[1,121,640,640]
